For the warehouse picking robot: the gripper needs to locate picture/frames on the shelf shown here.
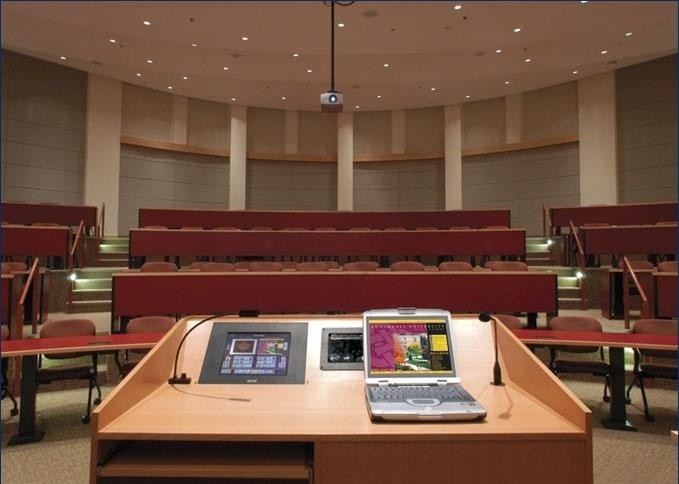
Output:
[579,226,679,269]
[577,225,679,267]
[508,327,679,431]
[0,331,168,446]
[1,275,20,336]
[1,268,33,324]
[0,203,98,237]
[0,226,73,270]
[603,268,679,320]
[110,272,559,325]
[548,202,677,239]
[129,229,527,269]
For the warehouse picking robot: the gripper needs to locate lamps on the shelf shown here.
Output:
[142,262,178,273]
[201,262,236,271]
[383,227,406,233]
[416,226,437,231]
[618,260,653,269]
[183,225,203,231]
[498,314,524,329]
[250,263,284,272]
[145,225,169,229]
[391,261,424,272]
[624,319,678,423]
[655,222,678,225]
[295,261,331,272]
[113,315,177,378]
[487,225,510,230]
[0,327,18,416]
[439,261,472,271]
[348,227,372,232]
[584,222,609,227]
[5,262,29,270]
[30,319,102,424]
[450,226,471,230]
[284,227,307,232]
[33,223,59,227]
[658,260,677,272]
[486,260,529,270]
[315,226,338,232]
[542,317,611,403]
[344,259,381,272]
[216,226,239,230]
[252,226,273,230]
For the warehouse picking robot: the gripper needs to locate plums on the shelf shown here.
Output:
[319,91,343,113]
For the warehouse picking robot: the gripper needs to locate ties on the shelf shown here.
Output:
[197,322,308,386]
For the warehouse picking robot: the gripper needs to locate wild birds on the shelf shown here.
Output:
[362,306,487,421]
